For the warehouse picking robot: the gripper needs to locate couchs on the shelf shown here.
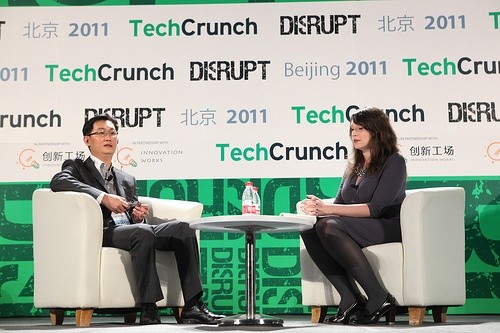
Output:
[296,187,465,327]
[33,188,202,327]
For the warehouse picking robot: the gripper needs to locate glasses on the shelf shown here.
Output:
[87,129,117,136]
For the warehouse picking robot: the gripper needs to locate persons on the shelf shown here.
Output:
[300,108,406,326]
[50,115,226,325]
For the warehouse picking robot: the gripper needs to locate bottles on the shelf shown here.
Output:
[253,187,260,215]
[241,182,256,215]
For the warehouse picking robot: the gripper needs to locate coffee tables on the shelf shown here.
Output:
[188,215,317,326]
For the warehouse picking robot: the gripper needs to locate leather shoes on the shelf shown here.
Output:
[140,303,161,325]
[181,303,226,324]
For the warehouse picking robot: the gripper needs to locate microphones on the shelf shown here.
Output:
[351,183,358,189]
[107,175,114,182]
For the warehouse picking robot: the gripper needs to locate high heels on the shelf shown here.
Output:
[351,292,395,325]
[325,299,361,324]
